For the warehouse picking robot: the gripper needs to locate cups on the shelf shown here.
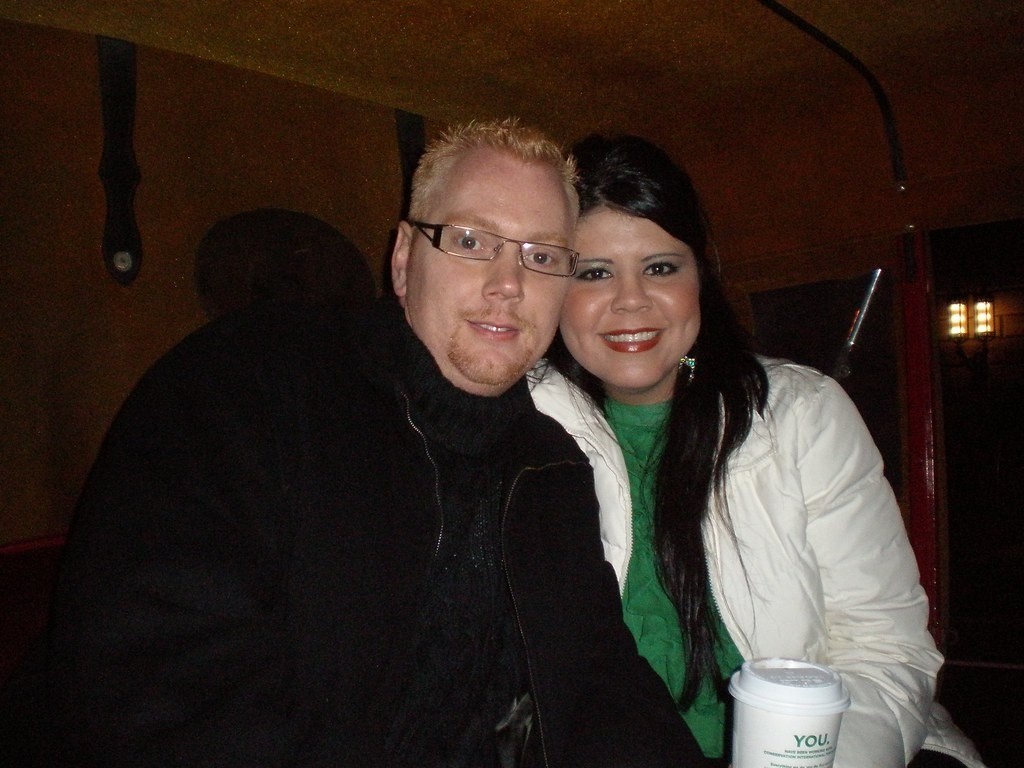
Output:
[727,657,851,768]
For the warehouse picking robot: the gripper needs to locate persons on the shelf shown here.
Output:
[0,114,700,768]
[523,128,985,768]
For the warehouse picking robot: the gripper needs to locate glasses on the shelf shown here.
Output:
[408,219,579,278]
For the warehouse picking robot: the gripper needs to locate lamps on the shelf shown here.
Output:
[946,294,969,343]
[972,295,997,342]
